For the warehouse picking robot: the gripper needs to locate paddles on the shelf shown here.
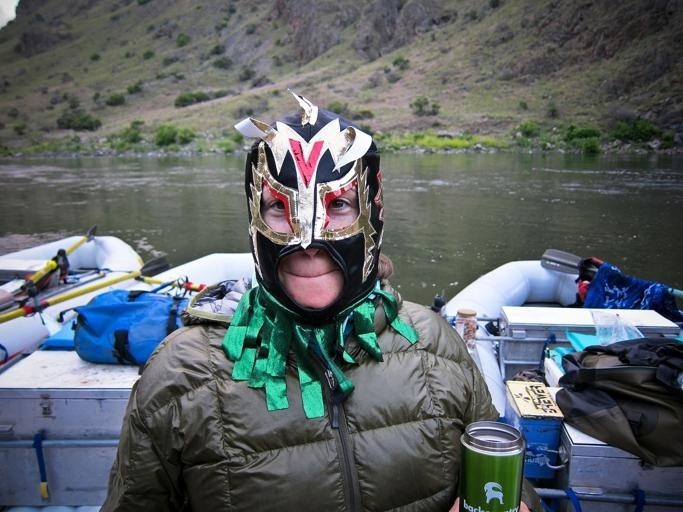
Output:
[0,254,170,325]
[541,249,683,298]
[20,223,97,293]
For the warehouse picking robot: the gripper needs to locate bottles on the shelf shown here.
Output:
[455,307,479,355]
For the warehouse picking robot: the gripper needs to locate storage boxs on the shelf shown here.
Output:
[0,350,146,512]
[497,305,683,512]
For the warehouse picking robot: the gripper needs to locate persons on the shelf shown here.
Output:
[97,88,545,512]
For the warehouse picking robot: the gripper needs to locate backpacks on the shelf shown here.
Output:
[74,290,190,364]
[555,337,683,467]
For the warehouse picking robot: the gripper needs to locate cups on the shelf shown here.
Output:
[458,419,528,512]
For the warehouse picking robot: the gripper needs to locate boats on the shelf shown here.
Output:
[436,256,681,512]
[0,251,261,510]
[0,232,145,372]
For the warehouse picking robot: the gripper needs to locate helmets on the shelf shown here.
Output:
[234,88,384,324]
